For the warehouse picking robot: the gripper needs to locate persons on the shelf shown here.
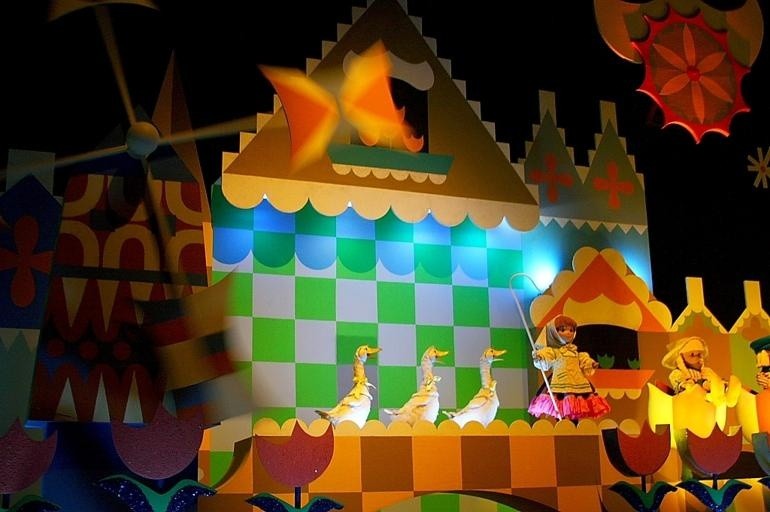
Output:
[527,315,612,423]
[661,330,728,395]
[751,333,770,390]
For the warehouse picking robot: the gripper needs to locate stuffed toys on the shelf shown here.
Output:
[383,344,449,424]
[315,341,382,430]
[443,346,506,429]
